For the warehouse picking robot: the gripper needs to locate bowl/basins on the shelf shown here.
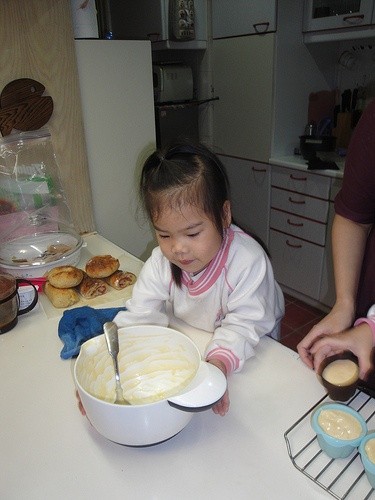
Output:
[299,135,336,152]
[0,230,88,279]
[73,323,229,446]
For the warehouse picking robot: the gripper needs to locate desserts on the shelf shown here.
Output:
[311,403,375,491]
[320,354,361,402]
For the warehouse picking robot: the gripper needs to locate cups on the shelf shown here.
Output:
[319,353,360,402]
[358,433,375,490]
[0,272,38,334]
[312,403,368,459]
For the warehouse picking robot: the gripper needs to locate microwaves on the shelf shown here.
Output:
[302,0,375,32]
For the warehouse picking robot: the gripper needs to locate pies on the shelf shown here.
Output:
[44,254,136,308]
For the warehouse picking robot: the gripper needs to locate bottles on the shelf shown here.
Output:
[306,124,314,135]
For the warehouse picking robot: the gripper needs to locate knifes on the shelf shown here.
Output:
[340,88,358,112]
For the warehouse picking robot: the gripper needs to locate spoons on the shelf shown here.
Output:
[103,321,130,405]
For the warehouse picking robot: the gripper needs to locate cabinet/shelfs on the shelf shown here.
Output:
[109,0,375,314]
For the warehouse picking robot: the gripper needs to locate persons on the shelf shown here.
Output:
[74,138,284,417]
[295,98,375,382]
